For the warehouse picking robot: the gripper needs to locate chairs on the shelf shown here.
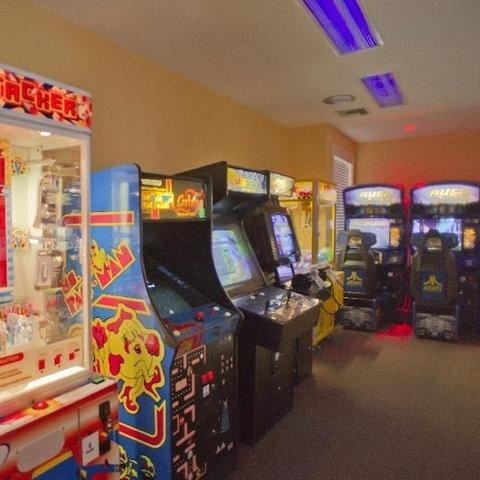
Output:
[407,229,461,342]
[335,230,387,332]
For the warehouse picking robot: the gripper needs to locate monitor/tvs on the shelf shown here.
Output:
[144,252,211,320]
[270,212,300,258]
[344,213,397,250]
[211,225,257,289]
[410,213,464,257]
